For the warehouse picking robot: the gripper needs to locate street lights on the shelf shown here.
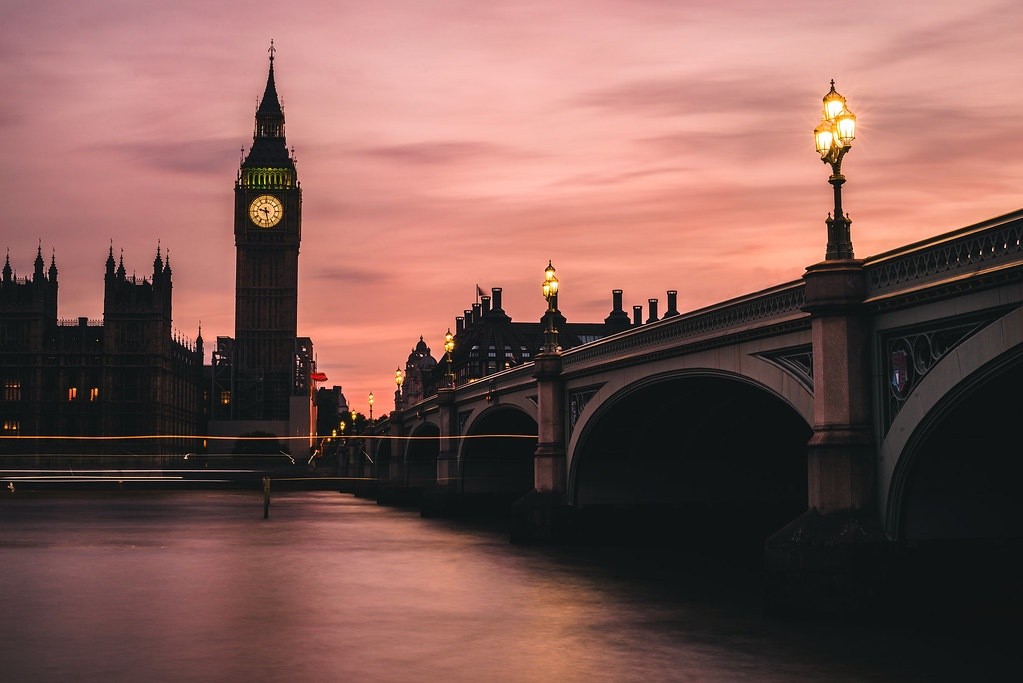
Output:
[332,429,337,446]
[538,259,560,352]
[351,409,357,436]
[394,366,403,410]
[369,391,373,419]
[812,78,859,261]
[340,420,346,445]
[444,327,455,388]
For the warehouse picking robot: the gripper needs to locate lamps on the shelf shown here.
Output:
[417,410,423,420]
[486,391,492,404]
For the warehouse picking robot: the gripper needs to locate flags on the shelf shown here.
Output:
[476,284,490,296]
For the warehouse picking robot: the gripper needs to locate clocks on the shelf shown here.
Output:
[248,196,284,228]
